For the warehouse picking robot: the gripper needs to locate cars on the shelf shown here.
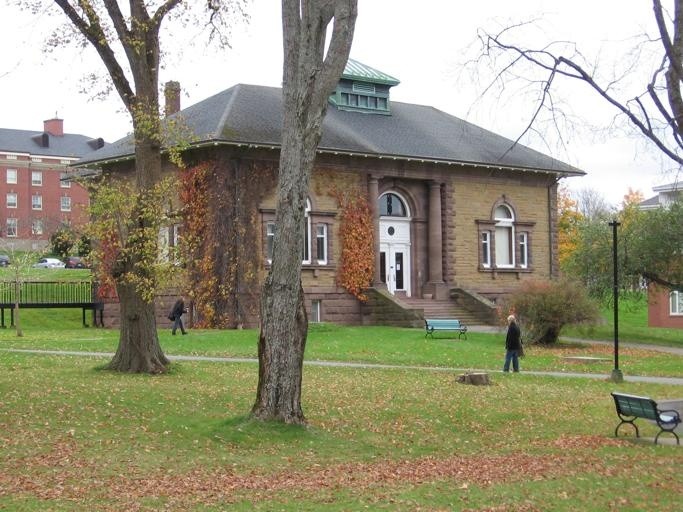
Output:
[66,256,86,268]
[33,258,65,268]
[0,254,11,267]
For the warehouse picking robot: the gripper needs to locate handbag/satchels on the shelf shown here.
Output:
[518,344,526,357]
[167,311,175,321]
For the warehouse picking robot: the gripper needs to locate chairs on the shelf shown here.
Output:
[610,392,681,445]
[425,319,468,340]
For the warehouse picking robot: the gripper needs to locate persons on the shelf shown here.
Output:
[170,295,189,335]
[501,313,520,373]
